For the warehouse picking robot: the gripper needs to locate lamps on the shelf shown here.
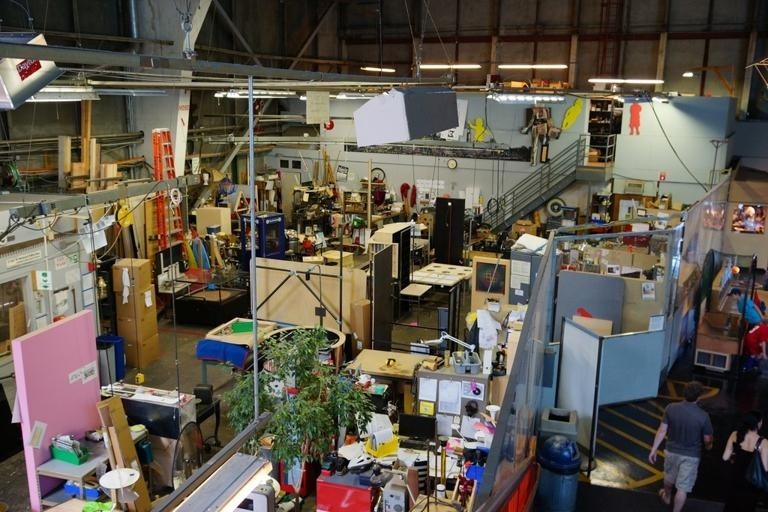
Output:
[1,32,64,112]
[212,91,227,98]
[171,452,274,512]
[335,92,377,100]
[616,95,656,103]
[225,90,300,100]
[24,86,102,104]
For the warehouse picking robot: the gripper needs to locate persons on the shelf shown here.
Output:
[217,176,238,197]
[726,287,764,330]
[739,324,768,374]
[648,380,714,512]
[722,410,768,512]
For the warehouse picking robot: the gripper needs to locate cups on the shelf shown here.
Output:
[433,484,446,498]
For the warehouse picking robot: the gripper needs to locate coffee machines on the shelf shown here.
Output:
[491,350,506,376]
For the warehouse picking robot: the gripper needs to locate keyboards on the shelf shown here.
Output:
[398,441,436,450]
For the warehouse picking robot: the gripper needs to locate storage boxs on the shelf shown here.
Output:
[125,333,160,370]
[112,258,152,292]
[117,306,158,343]
[510,250,543,306]
[452,351,482,376]
[115,284,156,318]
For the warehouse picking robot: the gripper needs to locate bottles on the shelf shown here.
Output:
[467,128,471,143]
[444,348,450,368]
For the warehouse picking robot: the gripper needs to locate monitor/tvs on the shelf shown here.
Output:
[398,414,436,440]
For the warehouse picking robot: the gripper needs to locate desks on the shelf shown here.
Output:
[399,283,434,327]
[372,206,408,229]
[347,346,434,413]
[173,268,249,330]
[194,317,277,384]
[412,261,473,351]
[298,233,331,246]
[240,210,285,271]
[35,428,151,512]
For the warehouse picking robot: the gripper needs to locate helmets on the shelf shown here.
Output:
[348,453,374,474]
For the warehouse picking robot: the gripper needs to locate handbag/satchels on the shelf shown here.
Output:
[744,437,768,488]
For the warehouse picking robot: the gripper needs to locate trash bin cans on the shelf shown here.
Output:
[96,341,116,386]
[96,335,125,382]
[539,407,579,443]
[536,434,581,512]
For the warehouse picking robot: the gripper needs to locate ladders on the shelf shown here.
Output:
[152,127,189,261]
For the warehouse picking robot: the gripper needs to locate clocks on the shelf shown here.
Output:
[371,168,385,182]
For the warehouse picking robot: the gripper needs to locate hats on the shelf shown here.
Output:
[726,288,741,296]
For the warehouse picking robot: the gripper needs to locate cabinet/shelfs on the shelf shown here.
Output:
[145,199,183,257]
[585,190,615,246]
[602,250,659,271]
[341,189,372,230]
